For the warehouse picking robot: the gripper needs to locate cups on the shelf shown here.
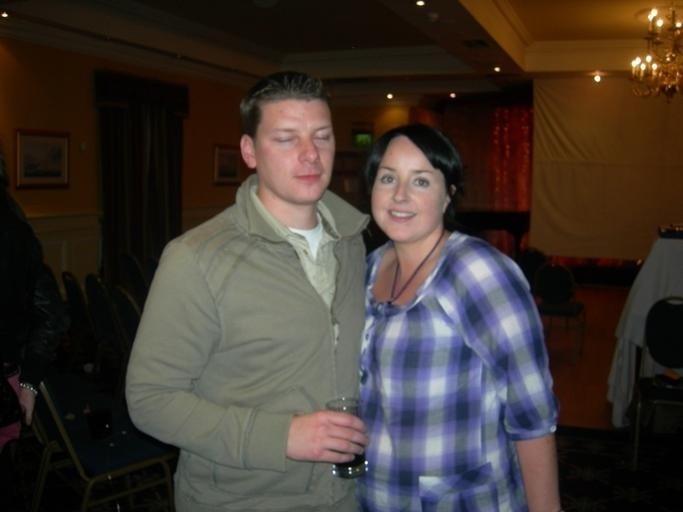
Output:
[327,397,369,479]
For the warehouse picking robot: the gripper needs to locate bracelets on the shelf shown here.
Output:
[17,382,39,397]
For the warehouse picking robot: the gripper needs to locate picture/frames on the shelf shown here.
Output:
[212,145,244,185]
[16,130,70,188]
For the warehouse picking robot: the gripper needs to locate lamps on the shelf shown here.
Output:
[630,0,683,104]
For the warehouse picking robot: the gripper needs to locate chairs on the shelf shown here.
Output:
[22,266,179,512]
[511,245,683,479]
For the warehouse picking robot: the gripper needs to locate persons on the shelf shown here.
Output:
[124,67,373,511]
[355,123,562,512]
[0,151,63,511]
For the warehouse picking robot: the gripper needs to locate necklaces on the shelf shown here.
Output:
[386,229,446,305]
